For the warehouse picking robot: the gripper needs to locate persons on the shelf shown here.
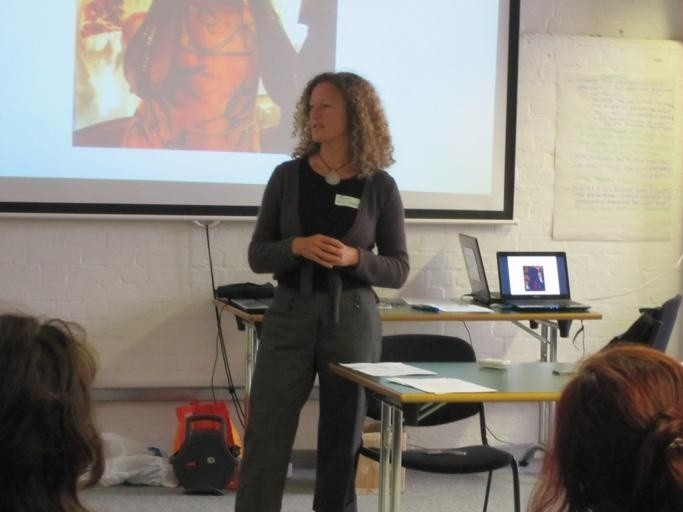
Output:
[526,344,683,511]
[1,311,108,510]
[231,71,409,512]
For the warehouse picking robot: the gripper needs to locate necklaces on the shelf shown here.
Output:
[316,151,353,187]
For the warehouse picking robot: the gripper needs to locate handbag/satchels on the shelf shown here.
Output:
[606,306,662,345]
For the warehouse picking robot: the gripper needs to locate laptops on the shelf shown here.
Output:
[457,232,502,305]
[229,297,275,313]
[496,250,591,312]
